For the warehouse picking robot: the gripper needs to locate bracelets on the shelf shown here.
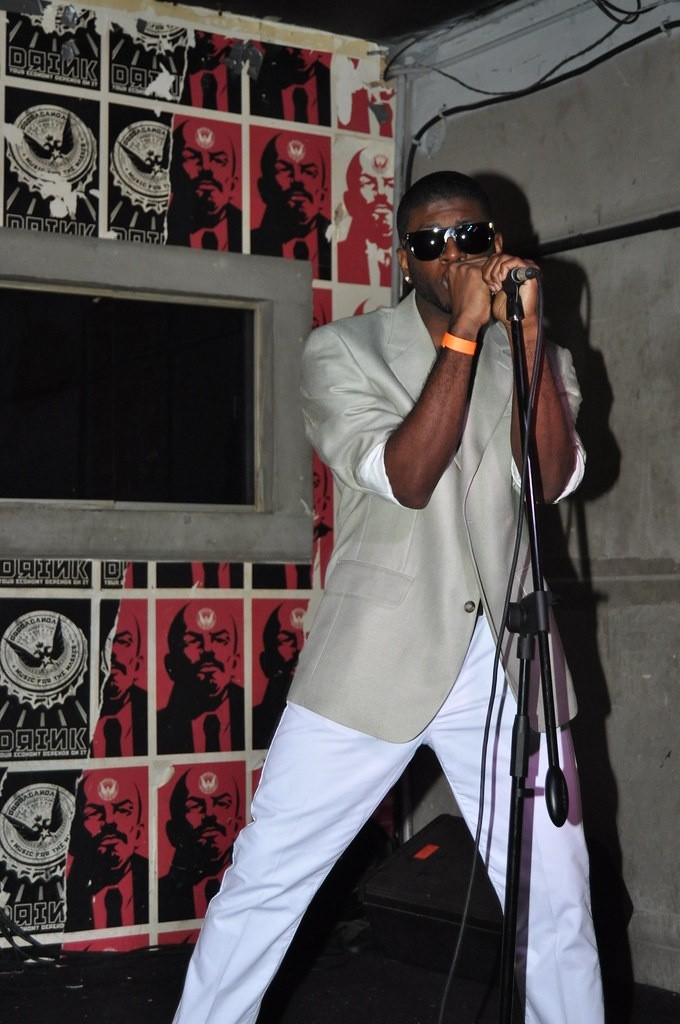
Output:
[440,331,477,355]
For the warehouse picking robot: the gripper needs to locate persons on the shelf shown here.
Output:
[172,170,604,1024]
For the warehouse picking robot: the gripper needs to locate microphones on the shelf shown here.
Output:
[499,265,539,286]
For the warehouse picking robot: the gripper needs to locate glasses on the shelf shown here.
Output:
[400,216,493,261]
